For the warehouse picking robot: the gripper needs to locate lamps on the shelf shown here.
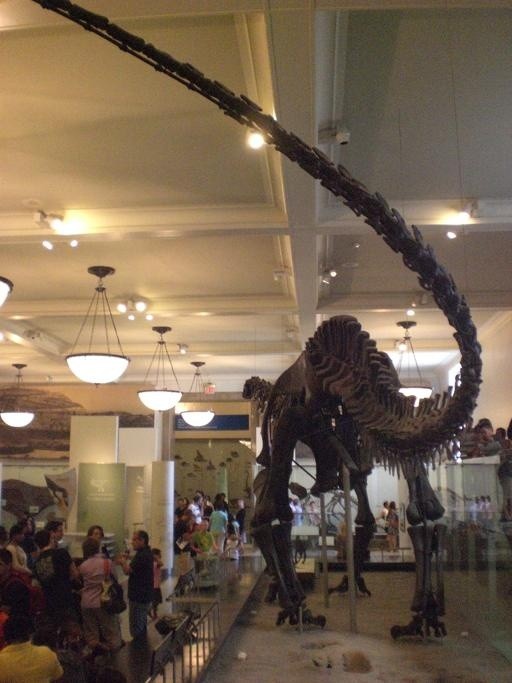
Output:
[0,363,38,430]
[65,265,216,428]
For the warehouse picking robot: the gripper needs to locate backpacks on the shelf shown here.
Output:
[100,558,127,615]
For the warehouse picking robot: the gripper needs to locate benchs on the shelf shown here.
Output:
[100,610,199,682]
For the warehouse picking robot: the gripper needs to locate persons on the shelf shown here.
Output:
[0,514,164,683]
[454,415,512,456]
[173,491,247,563]
[287,497,319,524]
[377,500,402,550]
[469,495,493,514]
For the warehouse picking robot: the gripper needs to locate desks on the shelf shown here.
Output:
[289,525,320,565]
